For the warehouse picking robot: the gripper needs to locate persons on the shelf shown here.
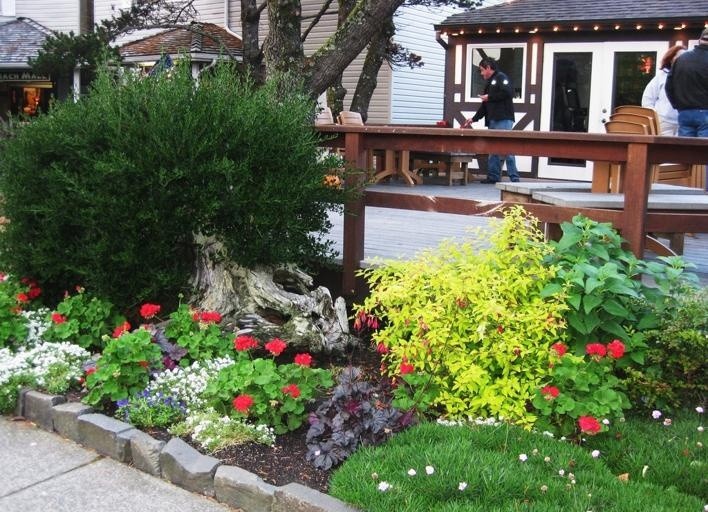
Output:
[465,57,520,184]
[641,44,688,136]
[664,28,708,137]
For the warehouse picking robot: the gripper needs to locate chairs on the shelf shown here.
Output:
[603,105,691,193]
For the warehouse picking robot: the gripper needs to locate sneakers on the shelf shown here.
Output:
[480,176,501,184]
